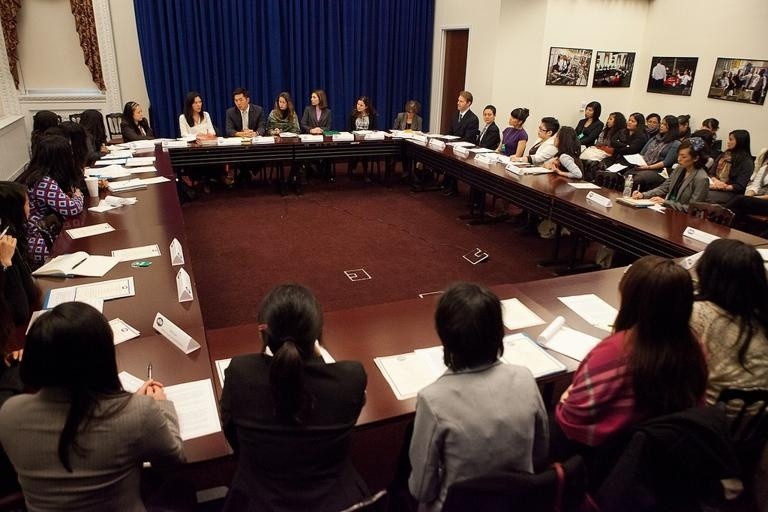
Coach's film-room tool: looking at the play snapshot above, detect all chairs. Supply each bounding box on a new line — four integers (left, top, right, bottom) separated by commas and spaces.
438, 455, 591, 512
104, 113, 123, 140
589, 401, 727, 512
706, 383, 766, 489
69, 113, 82, 125
684, 201, 736, 229
746, 214, 768, 224
321, 487, 402, 512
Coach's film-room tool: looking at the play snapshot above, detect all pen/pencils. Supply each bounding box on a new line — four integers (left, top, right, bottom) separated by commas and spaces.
72, 255, 89, 272
2, 224, 9, 238
147, 364, 156, 385
635, 185, 640, 197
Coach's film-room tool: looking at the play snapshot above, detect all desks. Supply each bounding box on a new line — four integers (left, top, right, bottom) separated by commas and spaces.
58, 147, 191, 232
31, 218, 234, 467
550, 188, 768, 279
108, 133, 294, 196
206, 280, 589, 467
405, 134, 495, 220
512, 243, 767, 413
289, 130, 422, 197
466, 155, 594, 266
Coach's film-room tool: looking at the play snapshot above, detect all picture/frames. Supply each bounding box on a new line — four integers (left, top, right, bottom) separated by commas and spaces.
646, 56, 699, 98
546, 46, 593, 86
707, 57, 768, 108
591, 50, 636, 88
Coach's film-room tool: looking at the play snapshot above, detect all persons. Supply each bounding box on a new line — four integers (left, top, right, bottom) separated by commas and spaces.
597, 112, 649, 191
496, 107, 530, 211
407, 283, 552, 512
22, 134, 85, 271
510, 116, 560, 234
707, 129, 754, 203
575, 101, 604, 148
347, 95, 379, 185
225, 87, 266, 191
219, 284, 373, 512
622, 114, 682, 191
555, 255, 711, 474
543, 126, 584, 179
645, 113, 661, 139
29, 110, 60, 161
0, 182, 37, 271
267, 92, 307, 198
721, 146, 768, 216
561, 54, 570, 70
677, 114, 692, 143
707, 57, 768, 106
674, 69, 693, 95
0, 347, 23, 368
585, 112, 627, 186
178, 90, 217, 196
300, 90, 336, 184
78, 108, 110, 157
650, 61, 668, 96
702, 118, 720, 142
439, 91, 480, 199
690, 129, 724, 176
48, 122, 90, 167
1, 301, 187, 512
632, 136, 710, 213
689, 238, 768, 420
468, 105, 501, 210
0, 226, 44, 326
120, 101, 156, 143
551, 55, 564, 76
393, 100, 422, 182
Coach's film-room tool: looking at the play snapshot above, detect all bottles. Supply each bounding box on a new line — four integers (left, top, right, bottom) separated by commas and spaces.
622, 174, 632, 199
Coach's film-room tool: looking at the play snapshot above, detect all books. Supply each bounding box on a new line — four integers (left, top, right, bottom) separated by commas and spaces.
32, 251, 118, 278
103, 180, 147, 195
